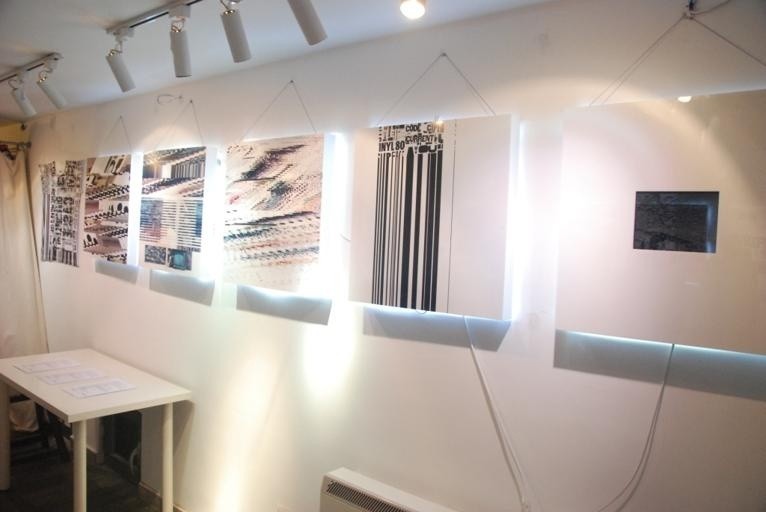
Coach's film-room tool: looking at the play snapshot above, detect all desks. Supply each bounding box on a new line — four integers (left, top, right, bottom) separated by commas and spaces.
0, 349, 190, 511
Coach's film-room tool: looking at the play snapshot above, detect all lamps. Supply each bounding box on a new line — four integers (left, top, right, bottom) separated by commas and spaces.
395, 0, 429, 21
99, 0, 329, 92
0, 51, 69, 119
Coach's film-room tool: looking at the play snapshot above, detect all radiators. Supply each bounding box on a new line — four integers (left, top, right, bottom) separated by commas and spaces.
317, 467, 457, 512
